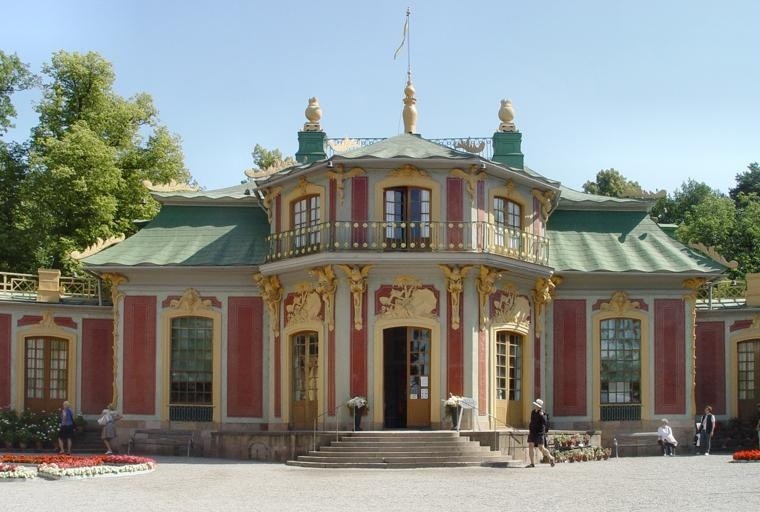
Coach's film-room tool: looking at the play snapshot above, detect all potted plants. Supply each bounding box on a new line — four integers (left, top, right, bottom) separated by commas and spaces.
553, 434, 611, 464
0, 408, 90, 452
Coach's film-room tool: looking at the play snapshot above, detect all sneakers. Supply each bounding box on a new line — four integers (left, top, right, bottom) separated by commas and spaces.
105, 451, 112, 455
550, 457, 555, 467
525, 464, 534, 468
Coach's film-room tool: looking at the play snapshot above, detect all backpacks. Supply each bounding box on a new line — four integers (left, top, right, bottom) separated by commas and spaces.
544, 412, 550, 433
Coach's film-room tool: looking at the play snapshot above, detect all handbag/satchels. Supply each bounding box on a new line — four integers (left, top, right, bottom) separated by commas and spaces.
59, 425, 77, 439
98, 416, 108, 426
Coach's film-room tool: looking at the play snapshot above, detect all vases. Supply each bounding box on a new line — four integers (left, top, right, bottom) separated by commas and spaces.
450, 405, 460, 430
352, 406, 362, 430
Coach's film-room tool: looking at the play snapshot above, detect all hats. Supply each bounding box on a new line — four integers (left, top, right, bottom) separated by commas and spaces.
533, 399, 544, 409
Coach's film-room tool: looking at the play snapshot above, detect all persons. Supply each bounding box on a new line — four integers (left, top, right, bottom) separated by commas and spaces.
97, 402, 117, 455
58, 401, 76, 455
655, 418, 676, 457
526, 398, 556, 468
697, 405, 715, 455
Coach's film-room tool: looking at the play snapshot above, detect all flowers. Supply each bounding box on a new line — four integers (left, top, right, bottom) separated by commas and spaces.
347, 396, 366, 407
440, 392, 461, 406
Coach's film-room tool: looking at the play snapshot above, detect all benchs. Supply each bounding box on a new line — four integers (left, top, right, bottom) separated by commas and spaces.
613, 432, 681, 458
128, 429, 195, 457
